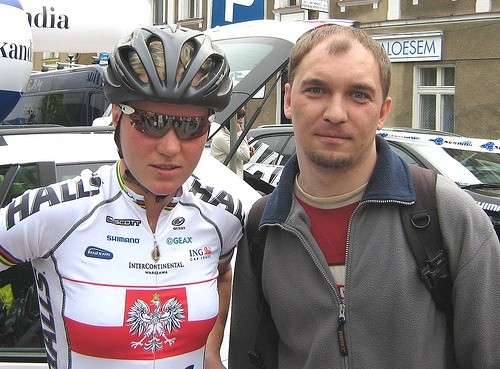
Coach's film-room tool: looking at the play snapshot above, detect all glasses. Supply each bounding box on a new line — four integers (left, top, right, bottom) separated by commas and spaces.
117, 103, 215, 141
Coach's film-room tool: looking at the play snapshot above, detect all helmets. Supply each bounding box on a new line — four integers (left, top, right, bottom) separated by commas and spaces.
104, 23, 232, 117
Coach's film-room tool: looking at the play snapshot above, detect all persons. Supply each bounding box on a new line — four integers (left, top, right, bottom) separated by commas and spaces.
227, 24, 500, 369
0, 24, 243, 369
210, 108, 256, 180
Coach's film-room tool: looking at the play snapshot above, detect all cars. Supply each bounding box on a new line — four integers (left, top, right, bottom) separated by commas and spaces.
246, 124, 500, 189
0, 18, 363, 368
1, 52, 111, 126
